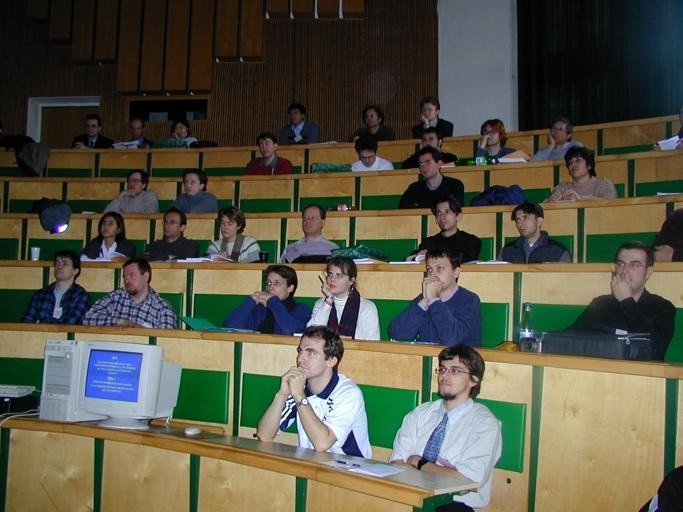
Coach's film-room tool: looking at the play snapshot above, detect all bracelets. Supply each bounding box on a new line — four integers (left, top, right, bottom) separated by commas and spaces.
324, 300, 332, 306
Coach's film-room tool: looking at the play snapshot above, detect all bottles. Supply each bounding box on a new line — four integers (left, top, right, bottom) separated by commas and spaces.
455, 157, 495, 165
520, 302, 534, 352
328, 203, 358, 210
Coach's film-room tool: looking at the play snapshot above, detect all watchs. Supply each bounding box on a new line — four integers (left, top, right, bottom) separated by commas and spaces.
296, 398, 309, 406
417, 456, 429, 470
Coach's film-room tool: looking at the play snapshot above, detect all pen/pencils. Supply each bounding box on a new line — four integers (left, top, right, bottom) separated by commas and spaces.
318, 275, 323, 283
334, 460, 360, 467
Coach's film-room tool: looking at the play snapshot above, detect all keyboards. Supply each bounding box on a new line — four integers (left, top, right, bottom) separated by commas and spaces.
0, 384, 36, 398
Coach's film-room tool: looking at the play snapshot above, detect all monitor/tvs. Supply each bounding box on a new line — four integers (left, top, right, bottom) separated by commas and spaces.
78, 341, 183, 430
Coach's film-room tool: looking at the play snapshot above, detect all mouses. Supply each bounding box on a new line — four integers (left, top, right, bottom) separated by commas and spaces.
184, 427, 203, 436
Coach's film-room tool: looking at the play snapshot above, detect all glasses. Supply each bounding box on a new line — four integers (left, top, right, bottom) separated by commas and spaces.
86, 125, 96, 128
265, 281, 281, 286
128, 179, 142, 183
435, 366, 472, 376
482, 129, 496, 134
614, 262, 644, 270
162, 220, 175, 225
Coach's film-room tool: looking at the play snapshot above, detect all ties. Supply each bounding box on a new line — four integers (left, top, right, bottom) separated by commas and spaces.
423, 413, 448, 463
90, 141, 94, 148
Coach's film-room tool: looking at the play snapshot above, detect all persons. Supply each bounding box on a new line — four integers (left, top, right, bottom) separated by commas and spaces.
71, 96, 682, 262
79, 257, 178, 329
566, 241, 677, 362
257, 325, 372, 459
386, 238, 483, 347
306, 256, 380, 341
389, 342, 502, 512
20, 250, 90, 324
223, 264, 312, 335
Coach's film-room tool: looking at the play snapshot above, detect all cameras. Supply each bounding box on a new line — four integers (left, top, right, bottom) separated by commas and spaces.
519, 337, 542, 353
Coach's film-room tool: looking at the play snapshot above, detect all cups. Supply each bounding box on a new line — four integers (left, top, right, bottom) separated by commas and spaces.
30, 248, 40, 261
257, 252, 270, 263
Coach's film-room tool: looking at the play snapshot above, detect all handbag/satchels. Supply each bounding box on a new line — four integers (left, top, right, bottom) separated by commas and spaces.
331, 244, 388, 261
542, 327, 653, 360
470, 184, 527, 205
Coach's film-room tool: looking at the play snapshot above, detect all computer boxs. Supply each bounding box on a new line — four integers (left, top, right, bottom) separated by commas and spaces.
39, 339, 109, 422
